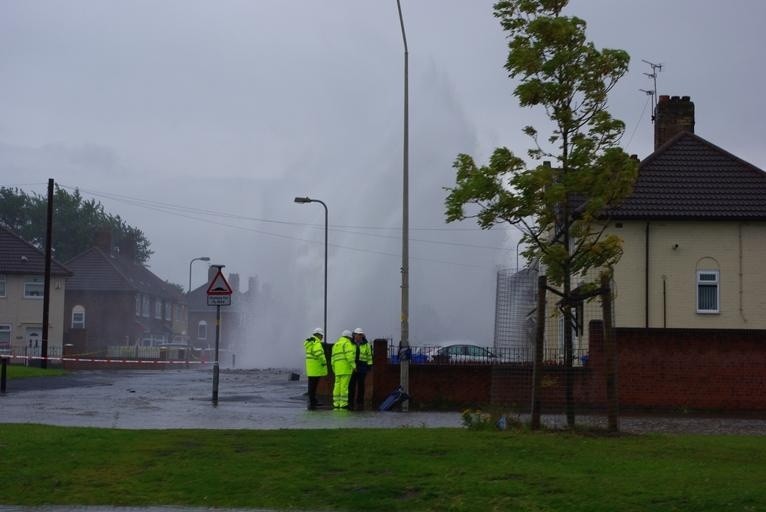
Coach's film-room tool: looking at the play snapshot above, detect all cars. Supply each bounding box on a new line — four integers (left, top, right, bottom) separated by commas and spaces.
422, 343, 517, 363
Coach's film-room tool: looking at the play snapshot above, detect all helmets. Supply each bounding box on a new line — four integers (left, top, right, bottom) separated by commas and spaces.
312, 328, 324, 336
342, 330, 353, 338
354, 328, 364, 334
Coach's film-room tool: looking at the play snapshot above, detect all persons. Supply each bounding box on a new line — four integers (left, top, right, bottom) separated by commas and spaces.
331, 329, 358, 409
303, 328, 328, 411
348, 328, 373, 411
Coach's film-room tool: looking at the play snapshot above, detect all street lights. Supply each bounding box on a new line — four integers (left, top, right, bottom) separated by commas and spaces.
185, 255, 210, 368
295, 197, 328, 347
